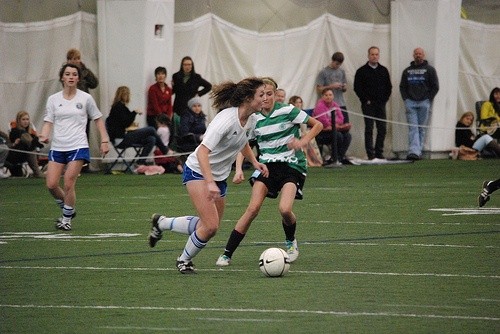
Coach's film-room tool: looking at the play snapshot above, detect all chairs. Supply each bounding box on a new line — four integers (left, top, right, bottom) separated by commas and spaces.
304, 109, 337, 164
169, 116, 197, 166
105, 117, 144, 176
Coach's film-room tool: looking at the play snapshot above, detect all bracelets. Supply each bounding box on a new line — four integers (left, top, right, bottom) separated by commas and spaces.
101, 142, 109, 143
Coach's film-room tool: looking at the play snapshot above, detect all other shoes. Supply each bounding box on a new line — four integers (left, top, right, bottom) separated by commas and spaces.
56, 212, 78, 229
61, 217, 73, 231
407, 154, 419, 160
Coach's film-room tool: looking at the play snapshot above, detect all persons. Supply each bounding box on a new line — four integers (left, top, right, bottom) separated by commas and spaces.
38, 63, 109, 230
456, 87, 500, 158
67, 48, 99, 172
477, 178, 500, 207
215, 76, 323, 265
353, 47, 393, 159
315, 52, 349, 123
4, 110, 49, 177
147, 56, 212, 161
148, 77, 269, 274
399, 48, 440, 160
274, 88, 351, 165
109, 86, 183, 174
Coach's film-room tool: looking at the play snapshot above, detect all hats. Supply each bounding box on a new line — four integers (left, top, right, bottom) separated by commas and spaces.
187, 96, 202, 109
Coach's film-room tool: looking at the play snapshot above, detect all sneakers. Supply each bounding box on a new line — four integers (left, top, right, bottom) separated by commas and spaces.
478, 180, 494, 207
176, 252, 196, 273
148, 213, 163, 247
285, 237, 299, 263
216, 253, 231, 266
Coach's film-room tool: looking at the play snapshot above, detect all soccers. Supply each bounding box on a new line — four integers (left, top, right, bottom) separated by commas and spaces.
259, 248, 290, 277
